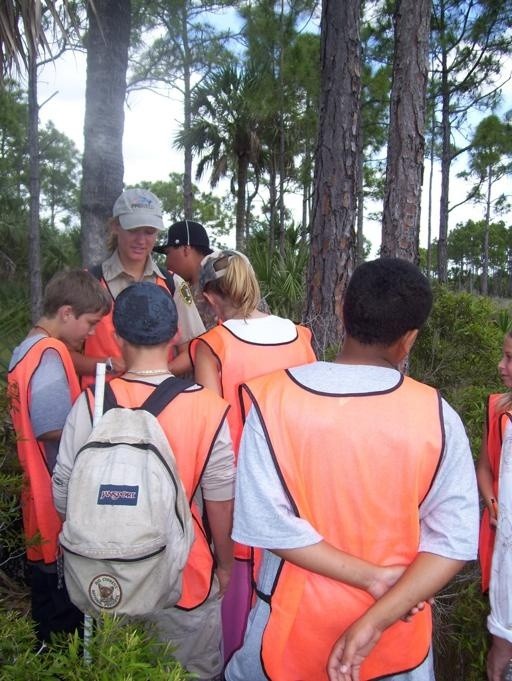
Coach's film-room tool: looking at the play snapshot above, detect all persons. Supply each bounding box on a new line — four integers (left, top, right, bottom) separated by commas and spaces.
188, 246, 317, 590
50, 281, 235, 680
223, 256, 486, 681
150, 218, 268, 329
9, 270, 111, 648
63, 189, 206, 390
475, 408, 512, 681
479, 332, 512, 594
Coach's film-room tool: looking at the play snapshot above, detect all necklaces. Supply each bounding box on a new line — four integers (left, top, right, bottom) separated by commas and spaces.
127, 369, 172, 376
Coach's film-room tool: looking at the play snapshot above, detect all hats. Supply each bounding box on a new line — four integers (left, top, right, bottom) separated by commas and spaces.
112, 188, 165, 231
153, 220, 209, 254
113, 282, 178, 344
199, 249, 250, 291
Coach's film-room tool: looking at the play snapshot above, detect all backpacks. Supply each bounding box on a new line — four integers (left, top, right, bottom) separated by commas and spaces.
57, 378, 195, 619
